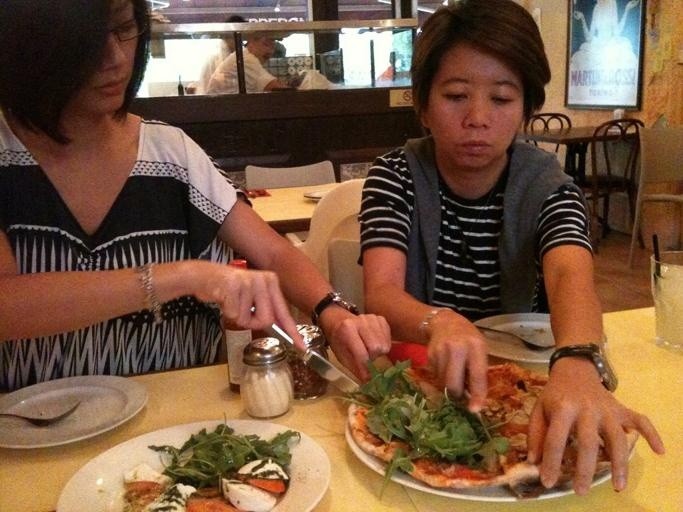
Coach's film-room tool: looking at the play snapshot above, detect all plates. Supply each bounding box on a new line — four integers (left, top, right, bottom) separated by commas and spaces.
301, 188, 329, 202
472, 311, 612, 365
3, 375, 146, 449
341, 421, 639, 504
47, 420, 331, 511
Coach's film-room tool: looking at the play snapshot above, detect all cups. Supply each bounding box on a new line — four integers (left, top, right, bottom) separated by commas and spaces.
648, 250, 681, 350
611, 109, 626, 129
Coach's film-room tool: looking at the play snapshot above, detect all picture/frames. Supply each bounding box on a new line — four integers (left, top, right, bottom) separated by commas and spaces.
562, 0, 647, 113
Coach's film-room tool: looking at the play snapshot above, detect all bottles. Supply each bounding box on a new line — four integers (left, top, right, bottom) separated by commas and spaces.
224, 258, 269, 391
284, 326, 330, 399
239, 339, 292, 420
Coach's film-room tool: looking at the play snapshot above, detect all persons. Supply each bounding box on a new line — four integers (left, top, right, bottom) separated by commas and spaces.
204, 30, 296, 92
196, 13, 247, 93
355, 2, 668, 498
0, 2, 393, 395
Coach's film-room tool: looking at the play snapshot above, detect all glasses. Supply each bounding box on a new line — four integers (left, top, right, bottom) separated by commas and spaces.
111, 15, 147, 42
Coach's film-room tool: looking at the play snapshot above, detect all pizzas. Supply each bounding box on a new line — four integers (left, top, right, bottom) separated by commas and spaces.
347, 361, 639, 488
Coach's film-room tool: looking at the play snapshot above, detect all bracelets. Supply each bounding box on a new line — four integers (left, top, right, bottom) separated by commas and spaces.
136, 259, 163, 311
415, 305, 452, 342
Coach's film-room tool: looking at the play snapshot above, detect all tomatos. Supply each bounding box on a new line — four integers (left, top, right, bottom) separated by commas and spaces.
185, 471, 285, 512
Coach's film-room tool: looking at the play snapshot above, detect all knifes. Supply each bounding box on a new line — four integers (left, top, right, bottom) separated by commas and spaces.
271, 327, 368, 398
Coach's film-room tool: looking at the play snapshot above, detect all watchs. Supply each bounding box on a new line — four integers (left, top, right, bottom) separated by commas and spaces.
311, 287, 360, 330
546, 341, 620, 391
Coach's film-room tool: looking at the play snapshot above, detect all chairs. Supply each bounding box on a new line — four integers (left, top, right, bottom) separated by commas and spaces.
627, 122, 683, 270
318, 231, 371, 316
521, 111, 574, 154
578, 117, 647, 257
282, 172, 371, 326
241, 159, 338, 193
